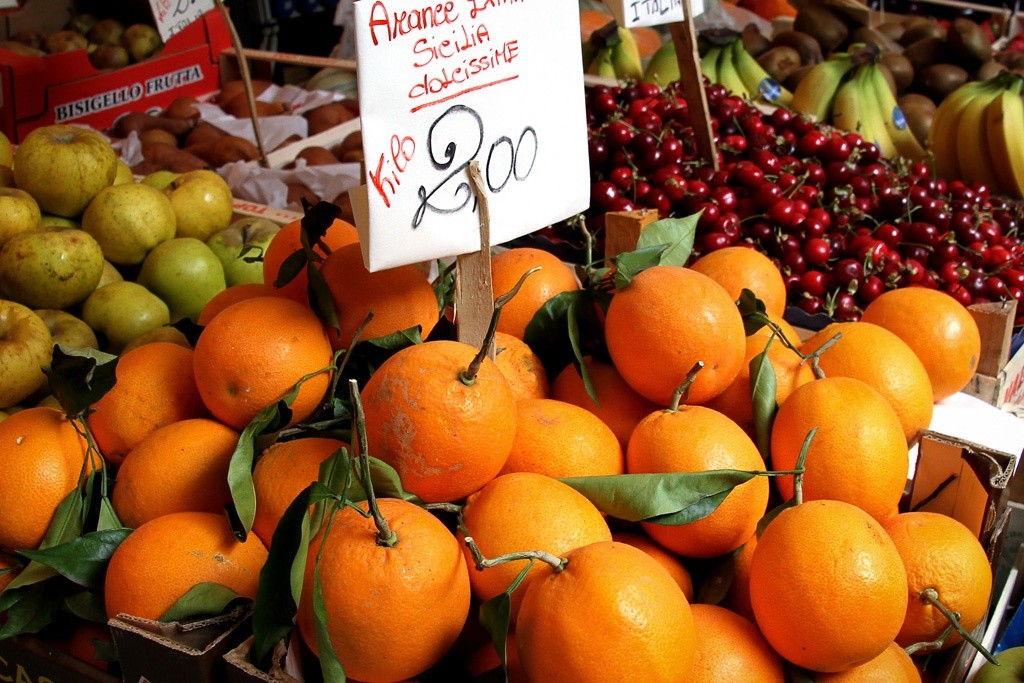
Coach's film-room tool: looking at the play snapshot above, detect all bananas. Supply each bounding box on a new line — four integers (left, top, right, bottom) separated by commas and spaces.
571, 8, 1024, 200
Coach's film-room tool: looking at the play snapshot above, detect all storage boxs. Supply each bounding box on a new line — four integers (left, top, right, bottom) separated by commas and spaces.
213, 111, 362, 228
0, 1, 234, 150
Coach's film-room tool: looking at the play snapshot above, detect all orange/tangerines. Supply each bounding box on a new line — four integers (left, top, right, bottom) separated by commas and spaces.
2, 208, 1010, 680
